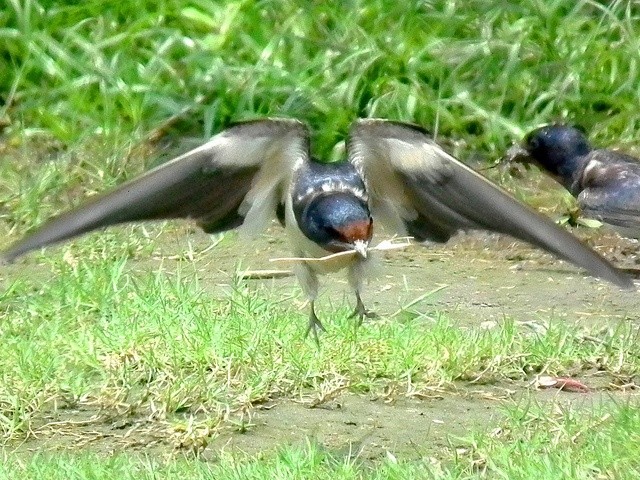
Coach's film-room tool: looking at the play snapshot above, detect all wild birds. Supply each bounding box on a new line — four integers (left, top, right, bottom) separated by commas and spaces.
0, 115, 635, 351
514, 123, 640, 241
